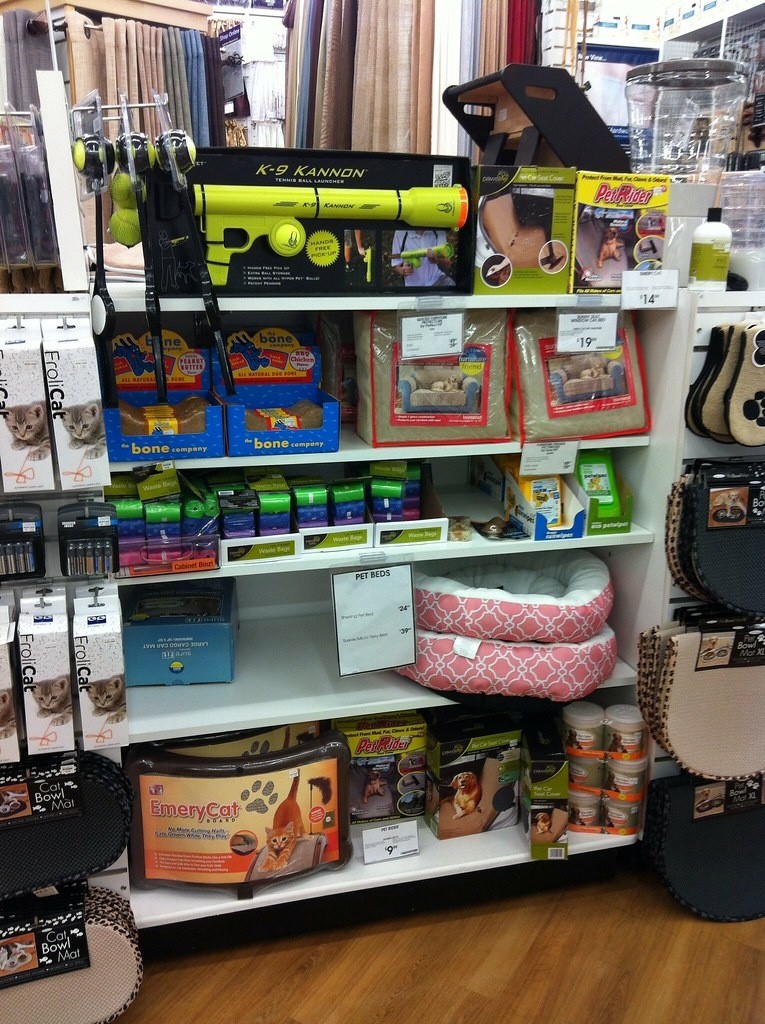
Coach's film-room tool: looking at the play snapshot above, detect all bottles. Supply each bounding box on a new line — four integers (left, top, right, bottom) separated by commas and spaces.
688, 207, 733, 292
562, 693, 646, 834
718, 160, 765, 292
625, 55, 748, 191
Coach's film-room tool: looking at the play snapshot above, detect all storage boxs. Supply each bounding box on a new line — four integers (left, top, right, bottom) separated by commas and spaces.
121, 577, 238, 687
469, 455, 632, 541
0, 584, 128, 765
118, 477, 449, 575
0, 317, 341, 493
330, 717, 570, 861
145, 146, 476, 298
461, 165, 670, 296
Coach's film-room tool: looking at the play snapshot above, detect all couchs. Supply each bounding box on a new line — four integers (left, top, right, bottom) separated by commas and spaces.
397, 369, 478, 412
549, 357, 622, 404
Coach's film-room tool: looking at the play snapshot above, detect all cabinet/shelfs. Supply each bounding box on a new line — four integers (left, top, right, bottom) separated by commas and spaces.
86, 283, 765, 928
